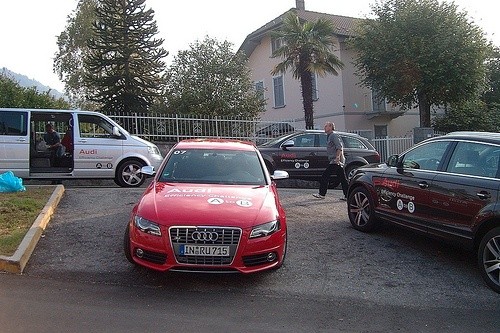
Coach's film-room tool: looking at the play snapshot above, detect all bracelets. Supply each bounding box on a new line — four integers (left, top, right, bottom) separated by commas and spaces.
49, 145, 50, 149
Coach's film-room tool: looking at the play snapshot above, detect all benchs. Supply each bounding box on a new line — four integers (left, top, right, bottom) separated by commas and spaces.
30, 131, 53, 167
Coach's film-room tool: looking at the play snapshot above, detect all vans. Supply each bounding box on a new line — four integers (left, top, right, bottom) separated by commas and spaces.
0, 107, 164, 187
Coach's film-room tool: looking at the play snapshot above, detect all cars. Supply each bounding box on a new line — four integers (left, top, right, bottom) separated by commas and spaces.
123, 137, 288, 279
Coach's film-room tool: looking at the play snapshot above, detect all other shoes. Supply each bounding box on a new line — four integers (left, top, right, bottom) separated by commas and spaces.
340, 195, 347, 200
313, 193, 325, 199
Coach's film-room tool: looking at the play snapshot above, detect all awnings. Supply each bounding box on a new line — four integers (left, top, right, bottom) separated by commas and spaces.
255, 123, 294, 135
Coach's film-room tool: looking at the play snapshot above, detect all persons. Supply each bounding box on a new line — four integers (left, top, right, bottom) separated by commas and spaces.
312, 122, 352, 201
39, 124, 62, 168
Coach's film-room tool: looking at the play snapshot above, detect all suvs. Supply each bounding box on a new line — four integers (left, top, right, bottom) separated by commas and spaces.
256, 130, 382, 190
343, 131, 500, 292
